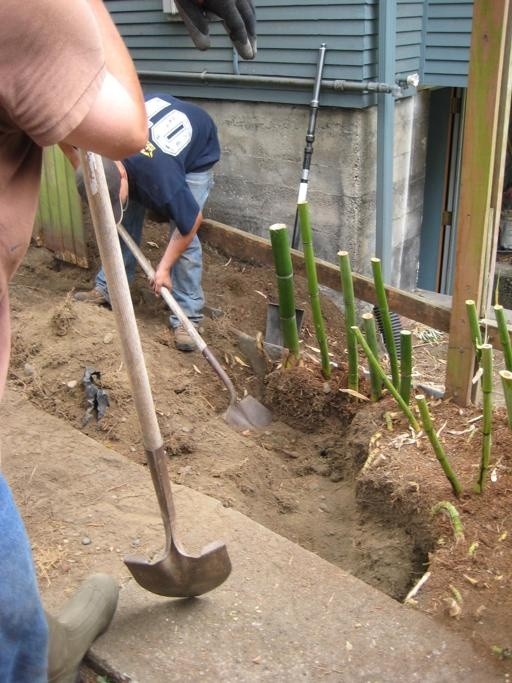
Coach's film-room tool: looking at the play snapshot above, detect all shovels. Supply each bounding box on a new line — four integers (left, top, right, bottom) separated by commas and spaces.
77, 146, 232, 598
115, 223, 274, 432
265, 43, 327, 350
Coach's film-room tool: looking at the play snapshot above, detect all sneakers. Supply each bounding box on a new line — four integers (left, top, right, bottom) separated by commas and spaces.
174, 322, 198, 351
75, 288, 105, 304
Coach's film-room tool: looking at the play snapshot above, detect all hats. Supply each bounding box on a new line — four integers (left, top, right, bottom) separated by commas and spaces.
76, 157, 123, 226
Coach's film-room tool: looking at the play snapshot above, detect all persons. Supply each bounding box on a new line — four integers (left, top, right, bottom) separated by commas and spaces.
75, 93, 222, 355
1, 0, 260, 683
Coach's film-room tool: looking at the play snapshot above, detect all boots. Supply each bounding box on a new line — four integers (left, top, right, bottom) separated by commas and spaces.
43, 574, 117, 681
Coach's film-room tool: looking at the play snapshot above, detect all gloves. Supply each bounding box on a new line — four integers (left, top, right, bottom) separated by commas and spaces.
173, 0, 257, 59
